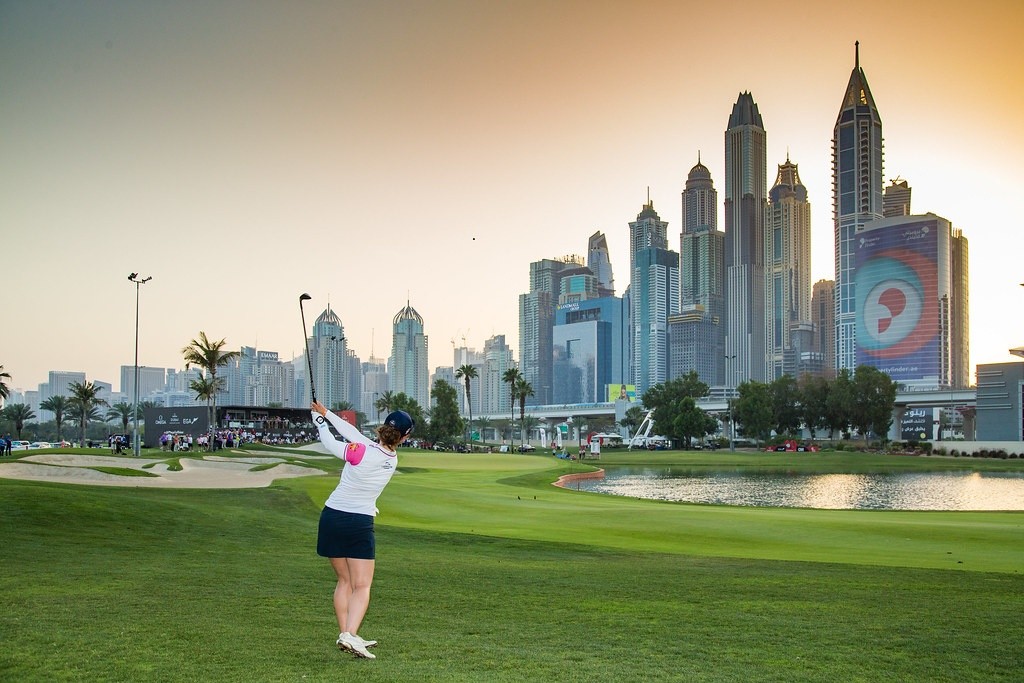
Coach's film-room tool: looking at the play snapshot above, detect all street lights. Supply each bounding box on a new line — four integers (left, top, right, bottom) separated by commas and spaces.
127, 273, 153, 457
331, 336, 346, 412
724, 354, 737, 451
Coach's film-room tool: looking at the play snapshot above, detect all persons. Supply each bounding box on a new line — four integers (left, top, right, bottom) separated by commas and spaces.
398, 438, 433, 450
579, 449, 585, 459
88, 441, 92, 448
80, 439, 84, 447
312, 401, 415, 659
341, 436, 350, 442
109, 433, 129, 454
370, 435, 378, 442
647, 441, 660, 450
566, 452, 570, 458
552, 448, 556, 457
160, 413, 320, 453
0, 432, 13, 456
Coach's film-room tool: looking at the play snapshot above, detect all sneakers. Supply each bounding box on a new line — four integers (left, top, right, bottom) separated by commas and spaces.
335, 632, 378, 649
338, 632, 376, 659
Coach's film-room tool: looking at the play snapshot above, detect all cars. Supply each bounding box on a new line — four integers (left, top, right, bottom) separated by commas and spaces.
4, 440, 62, 450
693, 440, 720, 450
518, 444, 536, 453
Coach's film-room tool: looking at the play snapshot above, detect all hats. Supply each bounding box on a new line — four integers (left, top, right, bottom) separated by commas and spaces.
384, 410, 416, 438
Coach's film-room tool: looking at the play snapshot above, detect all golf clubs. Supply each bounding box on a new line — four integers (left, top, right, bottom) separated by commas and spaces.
298, 294, 317, 402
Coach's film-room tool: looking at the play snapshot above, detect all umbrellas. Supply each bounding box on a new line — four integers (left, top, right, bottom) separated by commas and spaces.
635, 434, 679, 441
